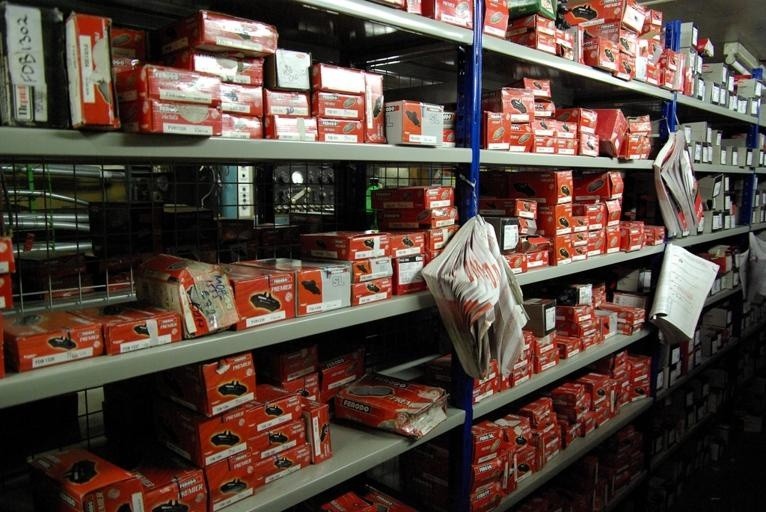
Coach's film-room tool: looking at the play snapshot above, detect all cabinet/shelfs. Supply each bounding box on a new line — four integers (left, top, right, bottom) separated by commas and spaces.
4, 1, 762, 508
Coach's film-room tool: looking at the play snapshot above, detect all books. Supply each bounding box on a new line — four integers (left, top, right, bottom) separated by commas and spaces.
652, 129, 703, 235
422, 214, 528, 381
738, 231, 766, 302
648, 243, 720, 348
